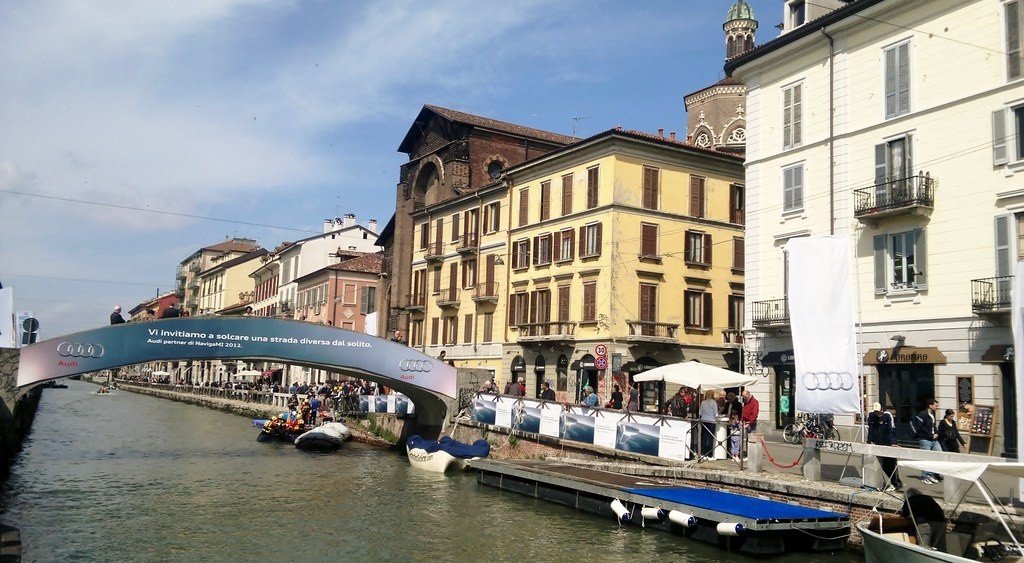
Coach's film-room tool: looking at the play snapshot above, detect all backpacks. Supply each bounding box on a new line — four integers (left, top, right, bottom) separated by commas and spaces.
907, 415, 923, 438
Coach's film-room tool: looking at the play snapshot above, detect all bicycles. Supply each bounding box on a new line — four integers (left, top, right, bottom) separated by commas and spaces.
783, 417, 841, 445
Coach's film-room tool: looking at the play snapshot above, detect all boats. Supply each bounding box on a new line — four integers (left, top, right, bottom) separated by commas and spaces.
854, 460, 1024, 563
256, 419, 491, 473
96, 386, 116, 394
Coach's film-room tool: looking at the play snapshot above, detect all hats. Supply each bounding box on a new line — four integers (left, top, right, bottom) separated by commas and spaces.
719, 390, 726, 398
873, 402, 883, 412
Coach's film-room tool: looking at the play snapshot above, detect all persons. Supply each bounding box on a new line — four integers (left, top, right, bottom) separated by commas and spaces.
478, 376, 640, 413
914, 399, 943, 485
867, 402, 903, 493
662, 387, 759, 461
901, 489, 947, 552
937, 409, 967, 453
110, 306, 456, 425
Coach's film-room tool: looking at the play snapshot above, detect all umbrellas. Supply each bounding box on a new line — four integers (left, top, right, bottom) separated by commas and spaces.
633, 359, 758, 418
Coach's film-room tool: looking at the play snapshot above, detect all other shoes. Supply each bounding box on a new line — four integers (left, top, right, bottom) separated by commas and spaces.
732, 457, 740, 463
703, 456, 716, 461
922, 476, 939, 485
886, 484, 903, 492
